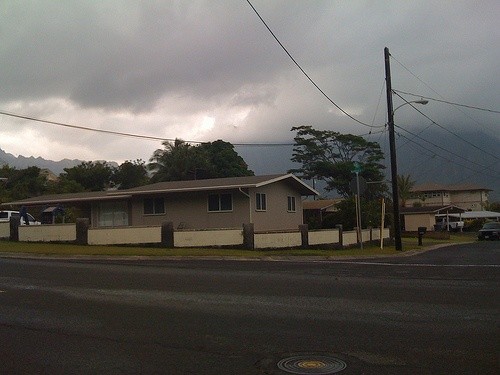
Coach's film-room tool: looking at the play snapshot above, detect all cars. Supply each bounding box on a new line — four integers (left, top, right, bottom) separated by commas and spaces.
477, 222, 500, 241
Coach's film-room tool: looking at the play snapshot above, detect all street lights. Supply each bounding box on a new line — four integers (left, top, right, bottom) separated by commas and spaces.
388, 99, 429, 251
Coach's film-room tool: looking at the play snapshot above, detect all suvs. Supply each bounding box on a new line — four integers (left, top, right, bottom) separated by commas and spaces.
0, 211, 41, 227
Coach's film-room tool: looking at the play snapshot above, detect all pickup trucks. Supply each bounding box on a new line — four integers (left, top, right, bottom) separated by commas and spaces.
436, 217, 465, 232
